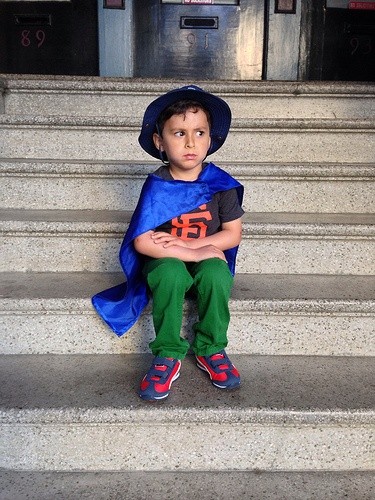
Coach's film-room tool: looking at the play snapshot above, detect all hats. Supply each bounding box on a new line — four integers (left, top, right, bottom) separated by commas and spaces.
138, 84, 231, 161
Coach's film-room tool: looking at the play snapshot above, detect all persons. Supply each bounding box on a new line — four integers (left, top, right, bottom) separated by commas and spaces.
91, 85, 245, 400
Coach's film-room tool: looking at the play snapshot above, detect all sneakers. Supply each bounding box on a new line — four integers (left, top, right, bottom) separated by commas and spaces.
195, 348, 241, 389
138, 356, 182, 400
